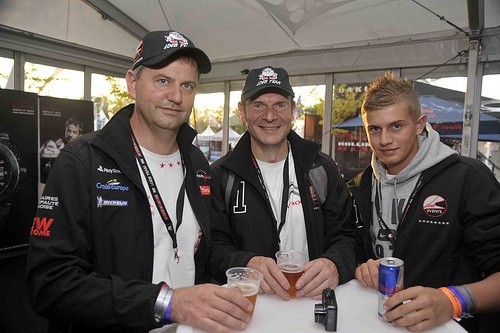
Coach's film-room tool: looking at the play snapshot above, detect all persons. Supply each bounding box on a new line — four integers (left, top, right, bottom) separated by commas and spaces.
347, 71, 500, 333
23, 29, 253, 333
40, 116, 84, 182
208, 65, 359, 300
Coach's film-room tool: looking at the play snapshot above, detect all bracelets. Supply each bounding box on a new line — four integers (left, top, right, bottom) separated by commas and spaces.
438, 284, 477, 322
153, 283, 175, 323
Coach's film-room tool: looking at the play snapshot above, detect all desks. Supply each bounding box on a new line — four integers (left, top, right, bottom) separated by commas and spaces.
174, 279, 471, 333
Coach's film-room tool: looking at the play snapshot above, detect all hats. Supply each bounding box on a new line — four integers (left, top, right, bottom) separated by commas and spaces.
241, 66, 295, 102
133, 30, 211, 74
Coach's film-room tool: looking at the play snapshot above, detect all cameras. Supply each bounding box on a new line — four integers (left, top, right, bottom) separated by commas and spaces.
314, 287, 337, 331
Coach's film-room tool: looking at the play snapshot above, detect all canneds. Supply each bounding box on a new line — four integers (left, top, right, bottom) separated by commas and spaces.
378, 257, 405, 319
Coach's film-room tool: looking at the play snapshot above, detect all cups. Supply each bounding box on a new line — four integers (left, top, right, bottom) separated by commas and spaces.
225, 266, 265, 318
275, 250, 308, 300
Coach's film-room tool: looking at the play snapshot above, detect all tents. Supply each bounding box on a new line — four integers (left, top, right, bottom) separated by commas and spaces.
193, 125, 240, 151
332, 95, 500, 164
